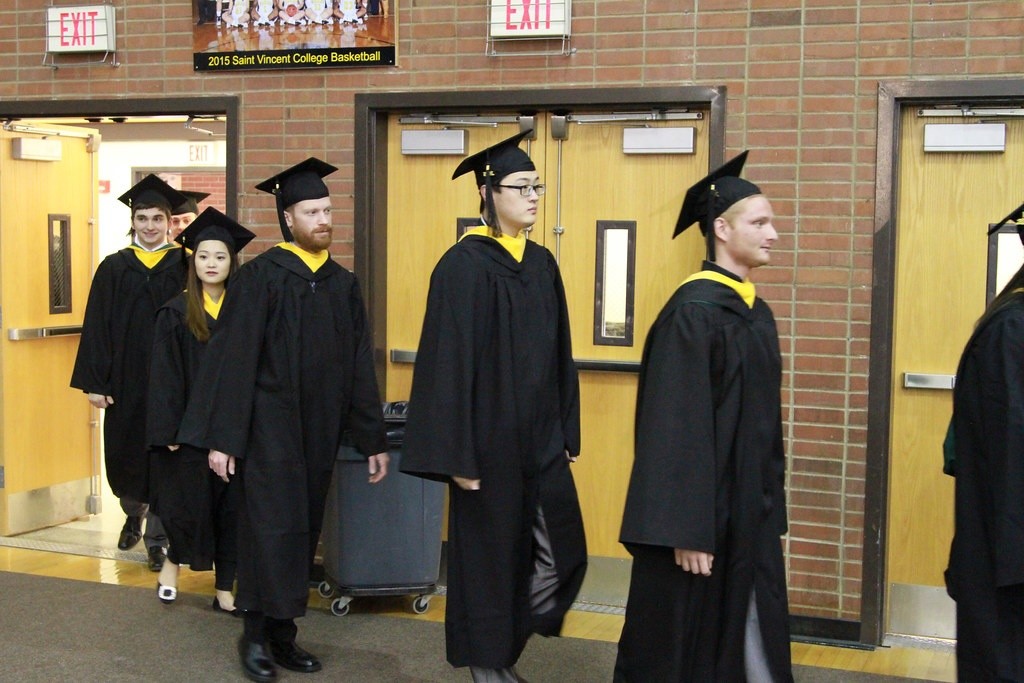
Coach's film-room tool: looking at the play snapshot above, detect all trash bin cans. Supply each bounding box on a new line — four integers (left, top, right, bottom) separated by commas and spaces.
328, 402, 446, 616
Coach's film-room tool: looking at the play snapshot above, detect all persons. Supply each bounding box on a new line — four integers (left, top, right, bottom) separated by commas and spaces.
943, 203, 1024, 683
398, 129, 587, 683
196, 0, 368, 29
612, 150, 795, 683
70, 173, 211, 573
178, 157, 390, 683
145, 206, 257, 618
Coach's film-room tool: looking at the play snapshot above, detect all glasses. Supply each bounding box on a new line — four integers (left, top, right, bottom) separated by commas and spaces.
495, 184, 546, 196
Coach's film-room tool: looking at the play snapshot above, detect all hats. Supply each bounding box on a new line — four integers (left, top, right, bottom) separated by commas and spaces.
254, 157, 338, 242
170, 190, 211, 216
452, 128, 537, 237
116, 173, 188, 212
987, 202, 1024, 245
173, 206, 256, 292
671, 149, 763, 262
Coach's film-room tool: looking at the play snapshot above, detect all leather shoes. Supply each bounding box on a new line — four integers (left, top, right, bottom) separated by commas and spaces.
268, 640, 321, 672
148, 545, 166, 571
211, 596, 248, 617
118, 514, 144, 551
156, 581, 178, 604
237, 634, 277, 682
